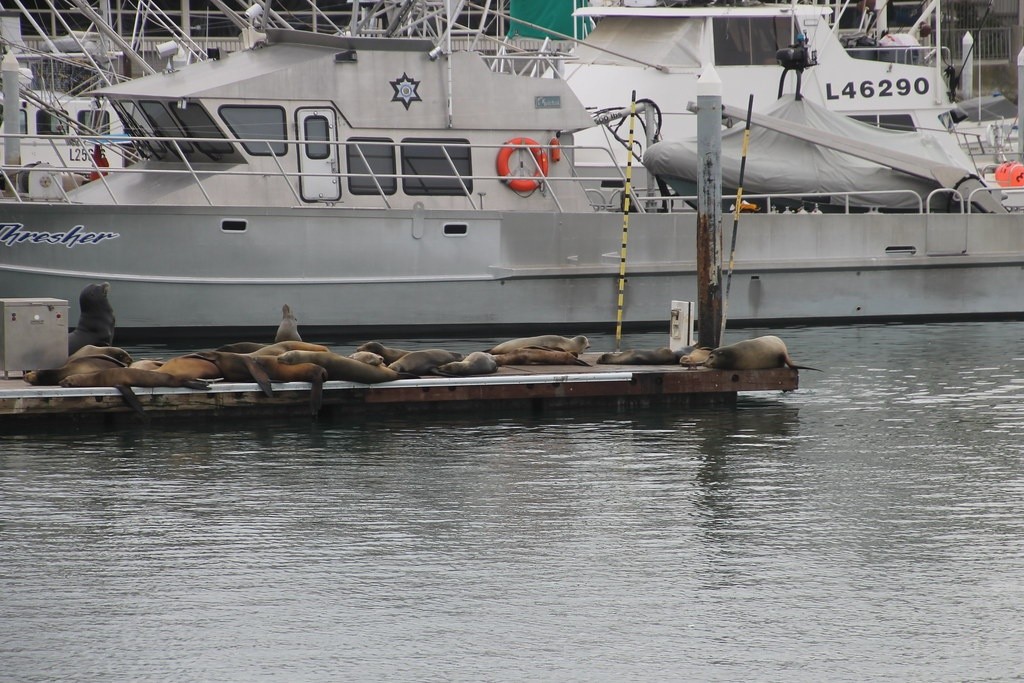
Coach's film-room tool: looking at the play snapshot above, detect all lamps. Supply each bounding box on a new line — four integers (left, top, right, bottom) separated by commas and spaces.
695, 62, 722, 96
155, 40, 180, 73
244, 2, 263, 29
428, 47, 447, 62
1, 50, 19, 71
177, 99, 187, 111
962, 31, 973, 45
92, 98, 101, 110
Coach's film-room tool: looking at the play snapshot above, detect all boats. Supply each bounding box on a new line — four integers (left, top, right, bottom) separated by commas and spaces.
0, 0, 1024, 340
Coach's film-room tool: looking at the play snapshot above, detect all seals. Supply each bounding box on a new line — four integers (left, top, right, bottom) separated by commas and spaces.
23, 280, 594, 416
597, 335, 825, 375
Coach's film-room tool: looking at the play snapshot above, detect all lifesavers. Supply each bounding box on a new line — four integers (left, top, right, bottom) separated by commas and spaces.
498, 137, 549, 193
